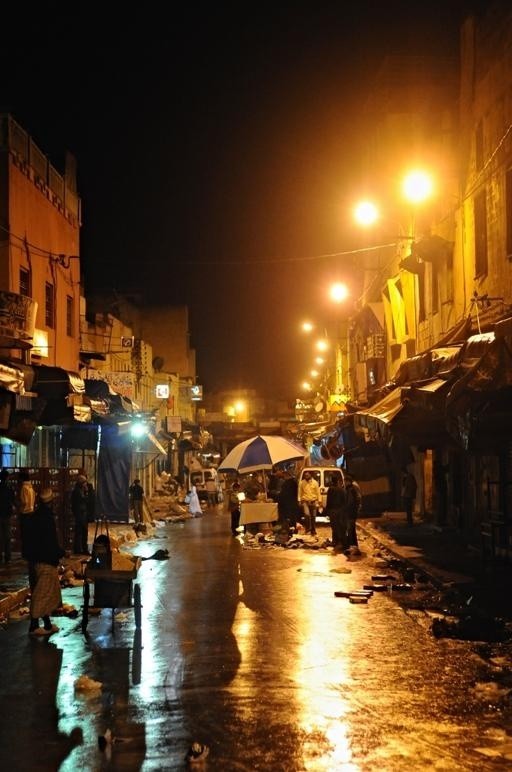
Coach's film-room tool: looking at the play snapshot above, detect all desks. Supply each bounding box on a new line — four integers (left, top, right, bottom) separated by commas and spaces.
237, 499, 284, 536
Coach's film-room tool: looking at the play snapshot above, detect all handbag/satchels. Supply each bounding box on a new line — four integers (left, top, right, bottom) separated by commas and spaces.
92, 535, 110, 568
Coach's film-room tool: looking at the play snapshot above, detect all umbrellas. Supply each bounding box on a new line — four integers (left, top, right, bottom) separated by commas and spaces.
216, 433, 308, 502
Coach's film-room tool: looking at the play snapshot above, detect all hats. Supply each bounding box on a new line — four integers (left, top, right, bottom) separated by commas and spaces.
78, 475, 87, 484
39, 488, 54, 503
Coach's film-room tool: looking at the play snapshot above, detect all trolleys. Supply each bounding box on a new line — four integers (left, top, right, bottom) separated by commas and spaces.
78, 514, 143, 635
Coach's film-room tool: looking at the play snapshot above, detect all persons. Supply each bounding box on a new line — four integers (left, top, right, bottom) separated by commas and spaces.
397, 466, 418, 527
1, 470, 21, 564
85, 483, 96, 523
226, 470, 363, 557
69, 475, 93, 555
129, 479, 146, 522
14, 471, 38, 559
22, 487, 73, 639
429, 448, 453, 528
158, 465, 220, 518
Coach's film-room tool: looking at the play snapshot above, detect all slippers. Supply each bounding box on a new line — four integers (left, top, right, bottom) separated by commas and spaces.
28, 624, 58, 634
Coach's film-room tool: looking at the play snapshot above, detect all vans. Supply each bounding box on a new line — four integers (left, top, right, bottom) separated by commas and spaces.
188, 468, 222, 504
297, 466, 347, 514
